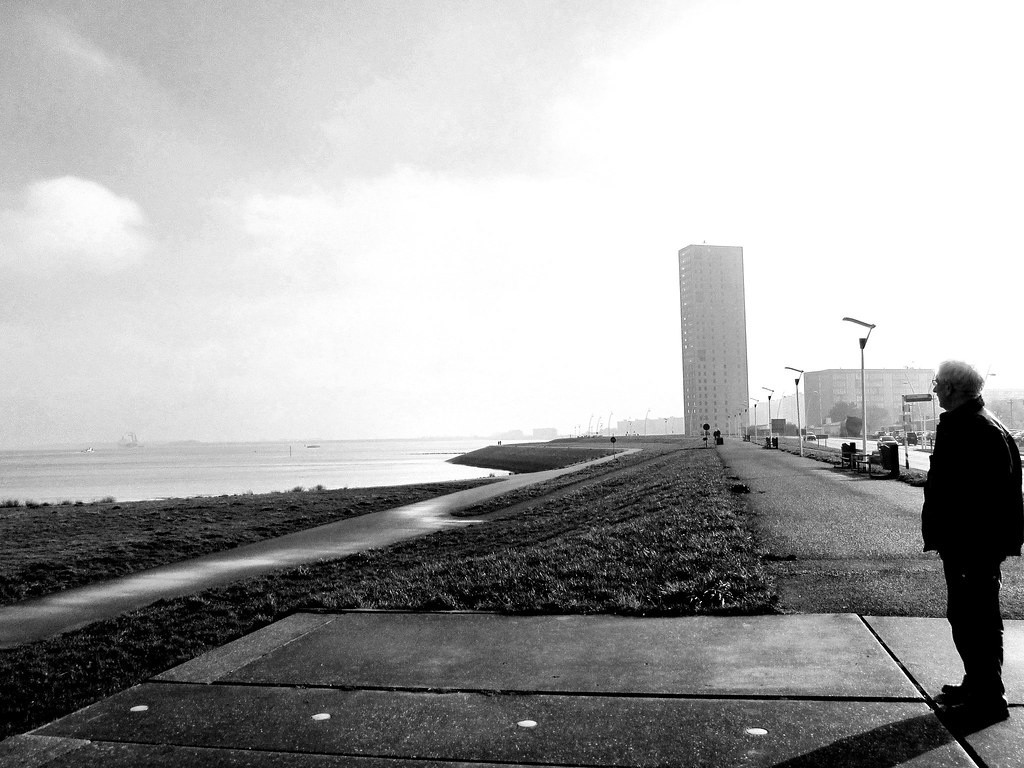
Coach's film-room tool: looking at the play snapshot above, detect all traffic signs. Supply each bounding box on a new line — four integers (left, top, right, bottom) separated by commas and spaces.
905, 394, 932, 403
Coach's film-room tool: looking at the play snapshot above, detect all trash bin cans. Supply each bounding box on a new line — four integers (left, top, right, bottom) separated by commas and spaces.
880, 444, 899, 474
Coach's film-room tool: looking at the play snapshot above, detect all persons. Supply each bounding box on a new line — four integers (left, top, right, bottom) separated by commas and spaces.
921, 361, 1024, 722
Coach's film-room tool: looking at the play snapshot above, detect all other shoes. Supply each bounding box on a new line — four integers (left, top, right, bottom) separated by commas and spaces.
942, 681, 976, 697
947, 697, 1010, 720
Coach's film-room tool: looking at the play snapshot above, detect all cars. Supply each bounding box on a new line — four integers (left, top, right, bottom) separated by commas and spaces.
892, 429, 937, 446
872, 431, 886, 439
877, 436, 899, 451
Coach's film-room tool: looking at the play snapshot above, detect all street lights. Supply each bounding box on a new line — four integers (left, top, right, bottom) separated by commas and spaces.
750, 398, 760, 444
841, 316, 877, 471
762, 387, 774, 447
784, 366, 804, 458
727, 402, 750, 440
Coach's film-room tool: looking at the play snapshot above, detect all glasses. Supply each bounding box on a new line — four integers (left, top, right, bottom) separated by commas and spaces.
932, 379, 942, 386
